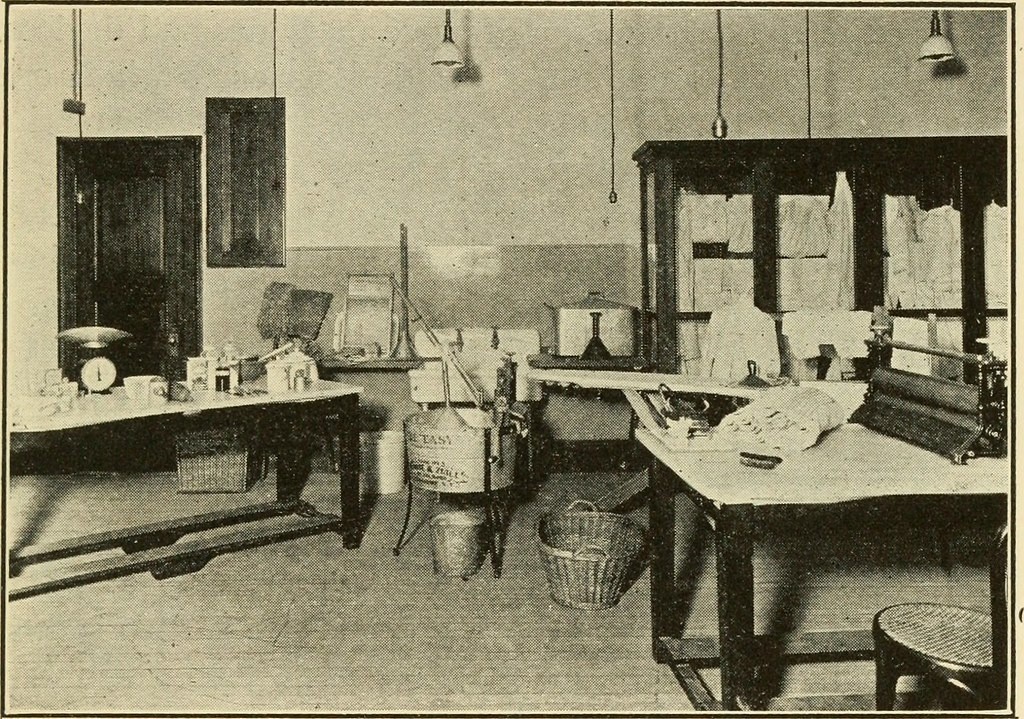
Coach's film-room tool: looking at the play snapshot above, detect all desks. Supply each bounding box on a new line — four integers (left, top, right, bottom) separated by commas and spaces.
9, 352, 1007, 711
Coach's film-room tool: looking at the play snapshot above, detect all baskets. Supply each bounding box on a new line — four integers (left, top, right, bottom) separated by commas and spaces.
174, 426, 250, 494
533, 498, 644, 610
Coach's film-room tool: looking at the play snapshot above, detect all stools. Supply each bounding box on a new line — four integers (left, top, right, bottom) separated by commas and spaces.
871, 601, 1008, 710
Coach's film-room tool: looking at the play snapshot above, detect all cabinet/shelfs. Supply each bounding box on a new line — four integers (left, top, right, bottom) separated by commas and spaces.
633, 134, 1008, 374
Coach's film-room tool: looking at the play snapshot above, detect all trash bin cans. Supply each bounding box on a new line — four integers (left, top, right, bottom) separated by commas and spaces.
431, 510, 484, 578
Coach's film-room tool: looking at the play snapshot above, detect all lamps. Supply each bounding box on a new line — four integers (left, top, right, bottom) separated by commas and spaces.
917, 10, 957, 62
431, 8, 464, 70
